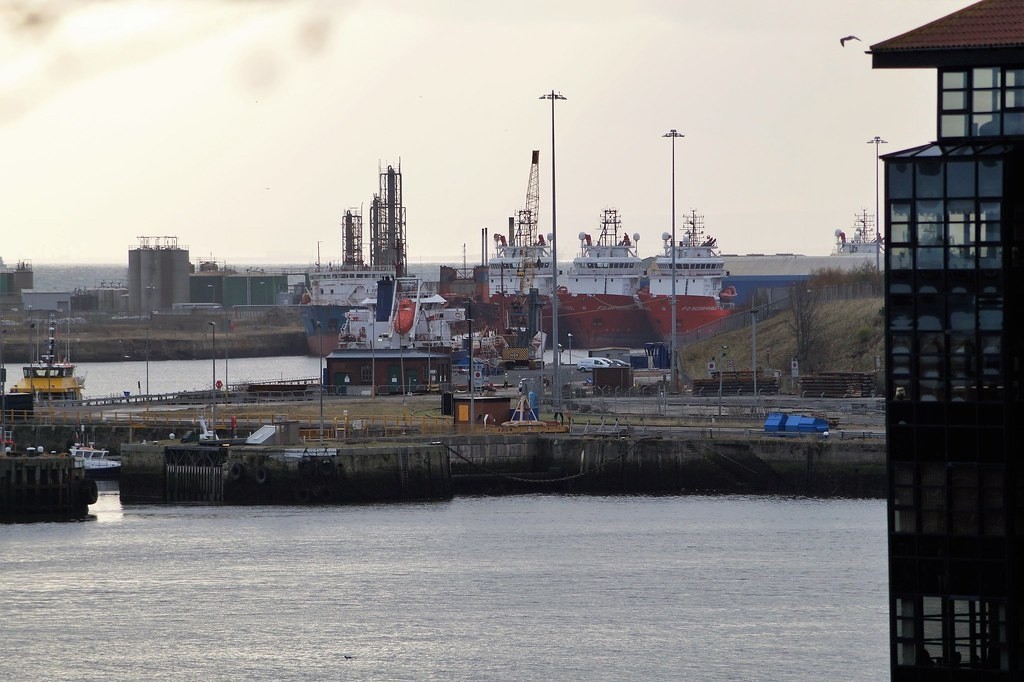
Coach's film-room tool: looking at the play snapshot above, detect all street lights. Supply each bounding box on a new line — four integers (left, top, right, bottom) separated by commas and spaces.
146, 308, 160, 401
314, 320, 325, 446
208, 320, 219, 442
661, 125, 686, 394
867, 133, 887, 271
750, 308, 759, 404
568, 333, 572, 365
539, 88, 568, 412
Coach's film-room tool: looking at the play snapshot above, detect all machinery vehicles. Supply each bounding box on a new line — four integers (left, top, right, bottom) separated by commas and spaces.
764, 411, 831, 437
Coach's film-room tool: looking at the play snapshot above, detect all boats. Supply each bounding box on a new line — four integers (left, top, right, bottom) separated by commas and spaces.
639, 210, 740, 341
482, 231, 559, 343
7, 305, 84, 405
331, 294, 469, 353
67, 443, 122, 478
297, 266, 448, 356
553, 205, 660, 349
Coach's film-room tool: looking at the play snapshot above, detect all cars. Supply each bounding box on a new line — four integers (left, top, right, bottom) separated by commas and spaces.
596, 357, 623, 368
519, 377, 535, 391
612, 359, 632, 367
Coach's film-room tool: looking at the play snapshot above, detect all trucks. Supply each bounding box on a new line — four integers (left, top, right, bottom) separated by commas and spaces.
577, 358, 611, 373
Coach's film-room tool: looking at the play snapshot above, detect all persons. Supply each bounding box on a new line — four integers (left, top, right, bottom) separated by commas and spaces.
504, 373, 509, 387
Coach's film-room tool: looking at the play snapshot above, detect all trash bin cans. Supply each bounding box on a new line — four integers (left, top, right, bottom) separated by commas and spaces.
785, 414, 802, 432
764, 412, 788, 432
799, 416, 830, 433
